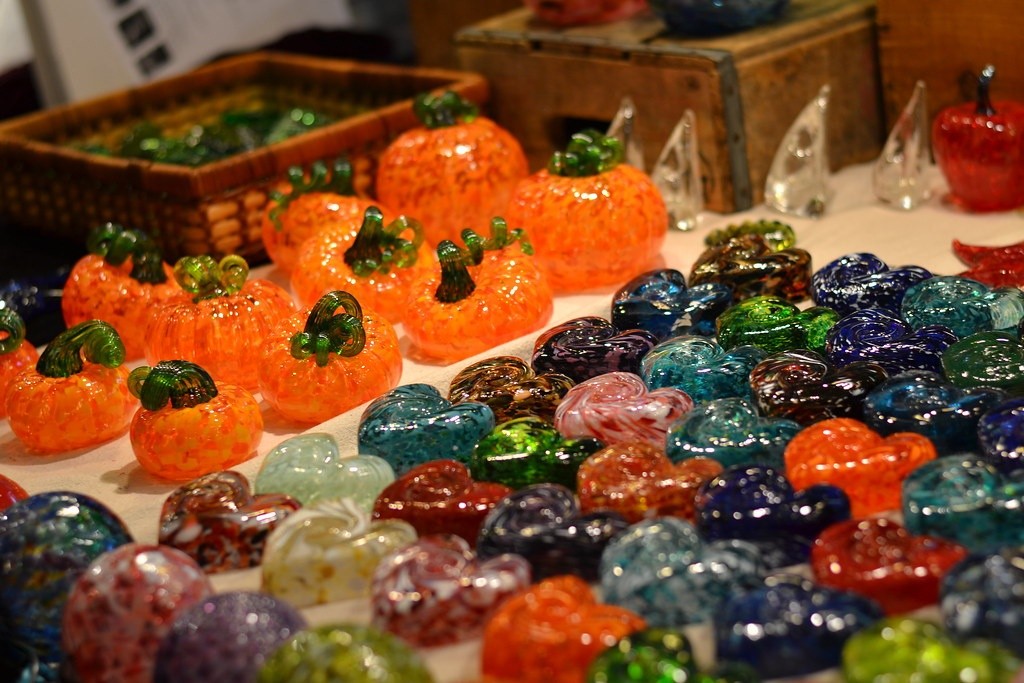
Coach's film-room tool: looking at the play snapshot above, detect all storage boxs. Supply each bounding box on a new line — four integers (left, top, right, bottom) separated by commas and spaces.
0, 0, 886, 271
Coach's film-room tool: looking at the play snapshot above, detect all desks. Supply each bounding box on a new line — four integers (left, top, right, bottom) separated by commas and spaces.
0, 159, 1024, 683
